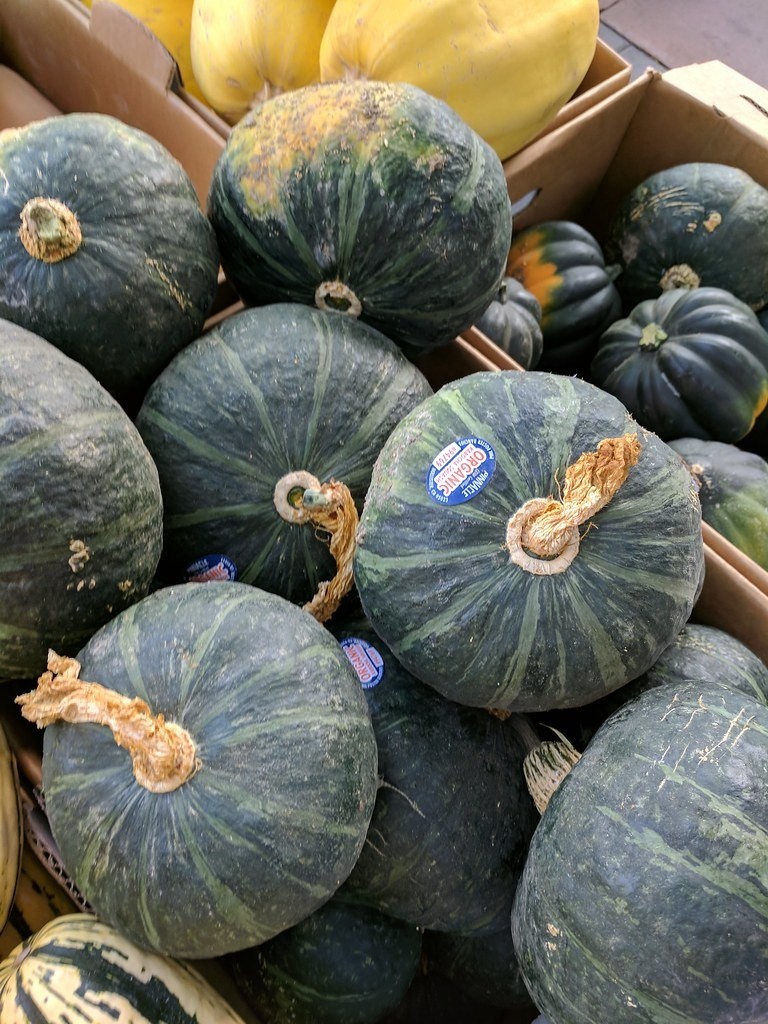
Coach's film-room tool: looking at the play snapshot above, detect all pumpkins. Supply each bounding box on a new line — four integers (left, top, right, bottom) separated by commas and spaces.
0, 0, 768, 1024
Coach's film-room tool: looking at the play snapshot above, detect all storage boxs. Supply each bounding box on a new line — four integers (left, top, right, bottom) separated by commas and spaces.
0, 0, 768, 914
